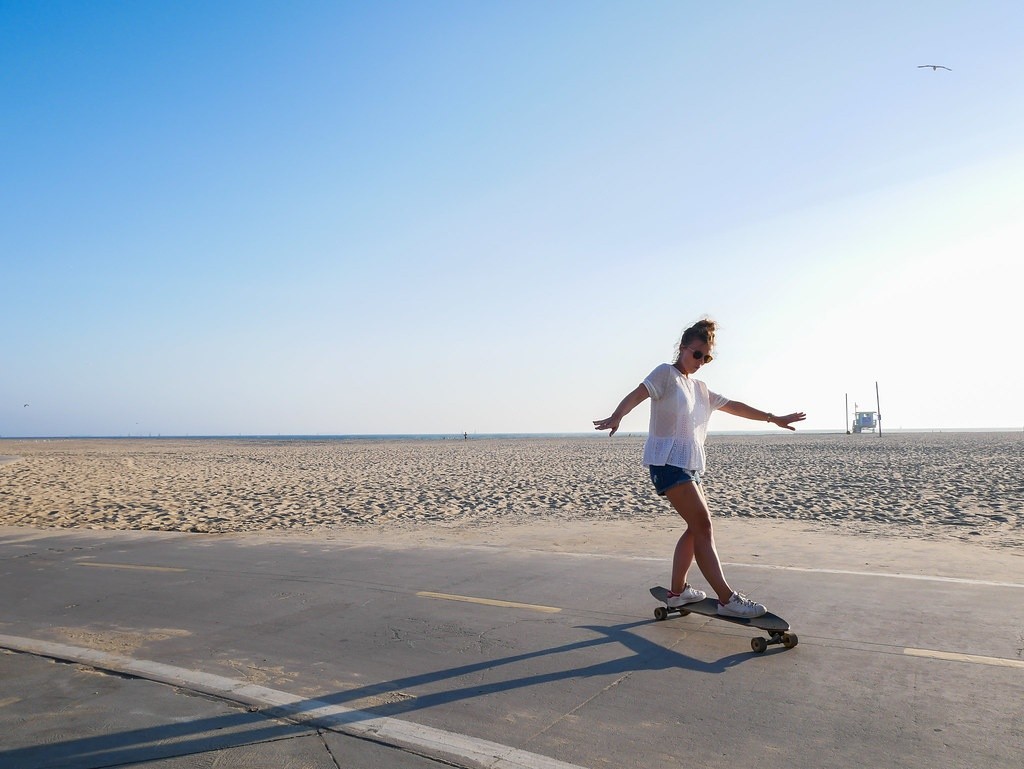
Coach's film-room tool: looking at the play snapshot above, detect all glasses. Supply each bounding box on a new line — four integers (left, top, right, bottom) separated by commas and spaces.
686, 346, 713, 363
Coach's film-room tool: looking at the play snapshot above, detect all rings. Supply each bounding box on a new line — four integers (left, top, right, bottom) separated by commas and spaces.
604, 423, 606, 426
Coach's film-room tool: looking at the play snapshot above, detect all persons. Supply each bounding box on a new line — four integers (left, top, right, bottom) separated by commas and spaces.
593, 319, 806, 618
464, 432, 467, 442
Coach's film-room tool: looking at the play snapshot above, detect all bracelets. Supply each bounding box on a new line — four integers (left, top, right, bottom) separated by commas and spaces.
767, 413, 772, 423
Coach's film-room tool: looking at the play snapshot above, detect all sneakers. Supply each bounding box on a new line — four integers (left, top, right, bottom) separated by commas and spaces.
717, 591, 767, 619
667, 583, 706, 608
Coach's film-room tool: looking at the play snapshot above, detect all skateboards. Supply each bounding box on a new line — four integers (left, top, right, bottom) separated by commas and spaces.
649, 585, 799, 653
